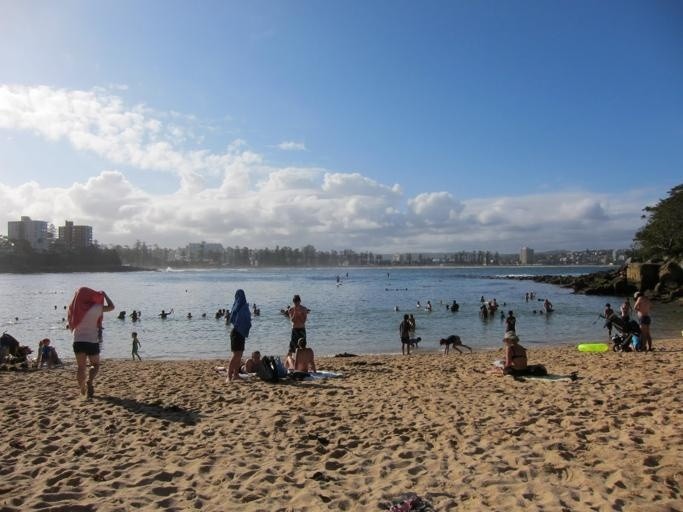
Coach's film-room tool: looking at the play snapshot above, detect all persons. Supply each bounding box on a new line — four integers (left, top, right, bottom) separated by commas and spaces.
213, 289, 318, 384
398, 313, 422, 356
497, 310, 528, 377
132, 332, 142, 362
439, 334, 472, 355
604, 291, 651, 353
119, 310, 142, 322
416, 290, 556, 320
68, 289, 115, 397
33, 338, 62, 367
159, 310, 172, 320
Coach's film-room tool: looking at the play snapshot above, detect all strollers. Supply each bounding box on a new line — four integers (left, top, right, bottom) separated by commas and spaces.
599, 313, 643, 352
0, 333, 31, 364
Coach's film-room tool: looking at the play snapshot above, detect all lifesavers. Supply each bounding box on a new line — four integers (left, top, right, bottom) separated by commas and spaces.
578, 343, 609, 353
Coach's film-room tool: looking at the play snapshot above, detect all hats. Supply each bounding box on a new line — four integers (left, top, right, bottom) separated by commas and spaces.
501, 330, 519, 343
